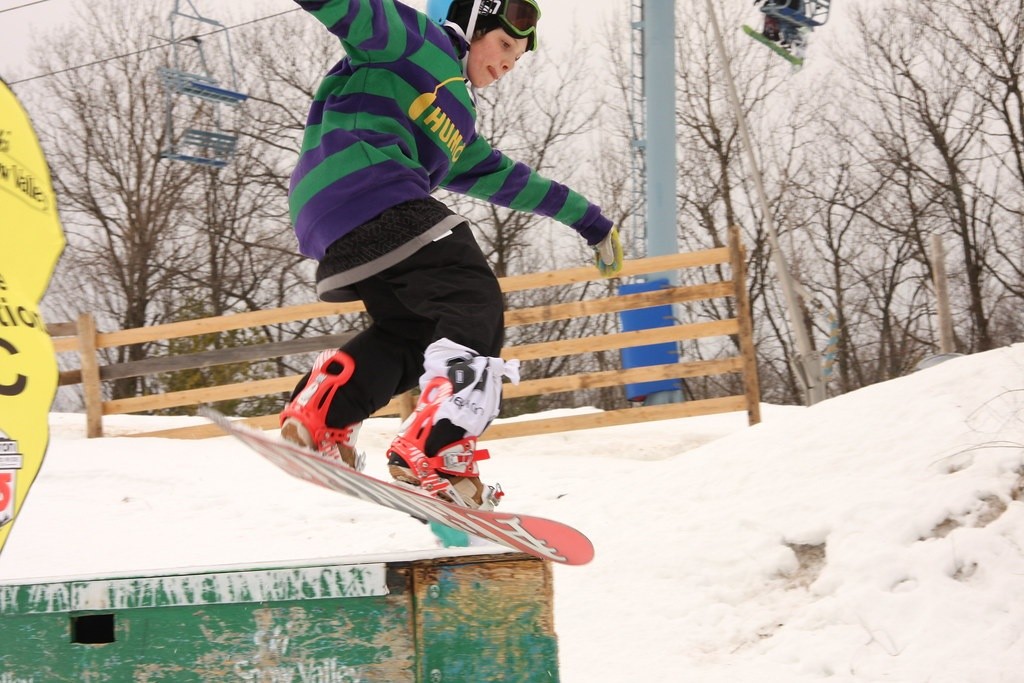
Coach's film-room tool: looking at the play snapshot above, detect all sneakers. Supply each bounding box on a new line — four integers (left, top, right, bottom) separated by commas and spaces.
281, 346, 367, 474
385, 376, 505, 512
780, 40, 801, 48
760, 29, 780, 41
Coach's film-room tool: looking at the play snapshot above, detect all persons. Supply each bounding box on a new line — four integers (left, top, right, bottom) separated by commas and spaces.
279, 1, 623, 512
753, 0, 807, 54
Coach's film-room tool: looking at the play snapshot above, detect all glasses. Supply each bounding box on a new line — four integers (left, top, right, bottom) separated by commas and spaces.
497, 0, 541, 52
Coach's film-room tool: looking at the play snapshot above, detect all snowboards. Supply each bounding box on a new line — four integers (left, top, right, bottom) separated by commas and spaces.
196, 405, 595, 569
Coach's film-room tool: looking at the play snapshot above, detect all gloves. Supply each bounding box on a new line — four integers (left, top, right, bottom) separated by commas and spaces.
590, 224, 623, 277
